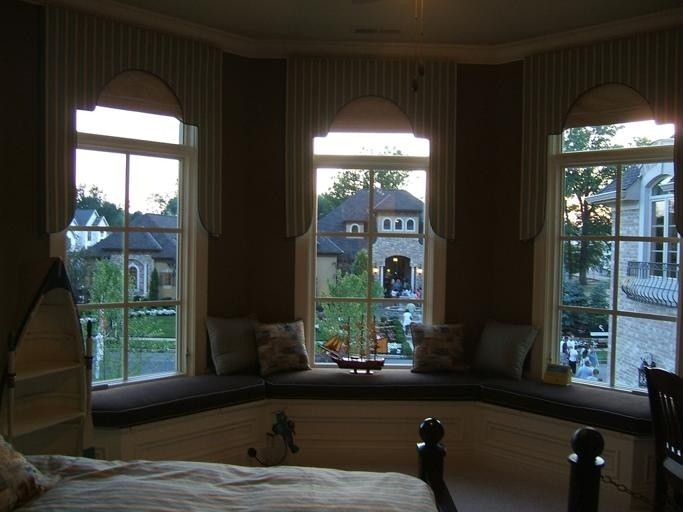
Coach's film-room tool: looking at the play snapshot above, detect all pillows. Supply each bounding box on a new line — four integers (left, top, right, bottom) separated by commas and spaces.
410, 323, 476, 374
256, 318, 316, 372
208, 312, 261, 374
0, 429, 47, 512
476, 324, 542, 382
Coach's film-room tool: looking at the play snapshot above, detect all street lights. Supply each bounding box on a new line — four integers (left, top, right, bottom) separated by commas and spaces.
373, 263, 381, 283
414, 265, 423, 294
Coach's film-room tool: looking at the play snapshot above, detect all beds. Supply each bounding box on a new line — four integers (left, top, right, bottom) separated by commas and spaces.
15, 420, 459, 512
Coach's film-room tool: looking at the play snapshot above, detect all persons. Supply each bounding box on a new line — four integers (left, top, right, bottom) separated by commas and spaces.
406, 301, 416, 317
559, 333, 602, 381
384, 268, 423, 310
402, 308, 413, 335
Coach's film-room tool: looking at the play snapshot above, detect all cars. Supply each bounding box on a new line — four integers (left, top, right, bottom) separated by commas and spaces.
131, 308, 176, 317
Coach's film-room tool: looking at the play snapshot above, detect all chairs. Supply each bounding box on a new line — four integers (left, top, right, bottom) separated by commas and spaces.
643, 365, 682, 512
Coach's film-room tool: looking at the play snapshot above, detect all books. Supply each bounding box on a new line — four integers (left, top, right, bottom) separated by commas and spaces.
458, 360, 489, 383
